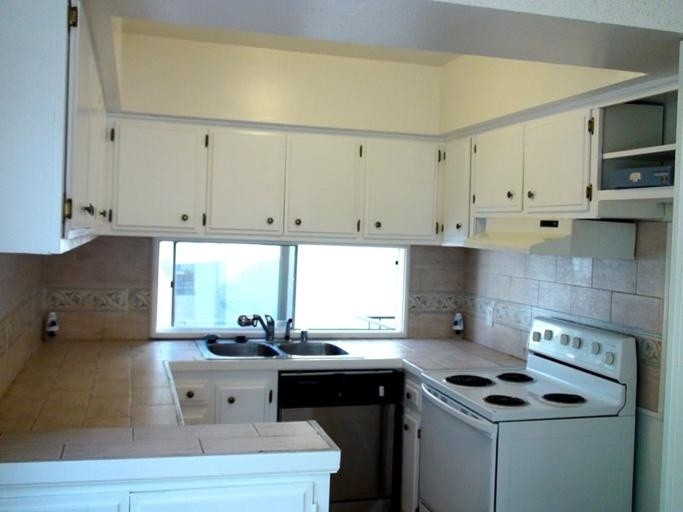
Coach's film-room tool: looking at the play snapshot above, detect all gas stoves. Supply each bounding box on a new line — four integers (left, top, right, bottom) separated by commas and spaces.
418, 353, 628, 424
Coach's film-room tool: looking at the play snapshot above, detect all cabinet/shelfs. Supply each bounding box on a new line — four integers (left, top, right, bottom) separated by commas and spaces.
112, 117, 201, 239
400, 379, 422, 512
62, 0, 108, 253
363, 135, 438, 246
473, 105, 665, 219
174, 378, 277, 427
440, 137, 471, 248
591, 82, 677, 201
0, 478, 321, 512
205, 124, 362, 246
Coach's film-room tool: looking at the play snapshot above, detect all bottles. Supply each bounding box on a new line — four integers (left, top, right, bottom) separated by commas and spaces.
451, 311, 464, 340
44, 311, 59, 342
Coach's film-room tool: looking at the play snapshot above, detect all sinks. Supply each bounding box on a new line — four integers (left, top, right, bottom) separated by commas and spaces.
266, 337, 361, 359
194, 336, 287, 358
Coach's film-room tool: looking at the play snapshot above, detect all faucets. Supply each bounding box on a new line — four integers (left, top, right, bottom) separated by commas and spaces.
253, 314, 275, 343
284, 318, 292, 339
